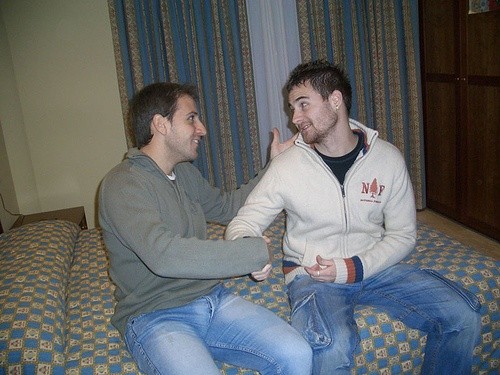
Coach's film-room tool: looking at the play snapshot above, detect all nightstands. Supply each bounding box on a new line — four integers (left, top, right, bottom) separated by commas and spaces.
8, 206, 88, 230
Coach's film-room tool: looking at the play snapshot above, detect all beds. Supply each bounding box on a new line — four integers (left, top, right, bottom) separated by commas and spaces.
1, 218, 499, 375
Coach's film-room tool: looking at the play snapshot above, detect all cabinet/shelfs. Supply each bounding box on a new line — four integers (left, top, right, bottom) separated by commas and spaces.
419, 0, 499, 240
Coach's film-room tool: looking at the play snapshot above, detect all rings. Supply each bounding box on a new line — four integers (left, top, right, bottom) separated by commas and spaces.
318, 270, 320, 277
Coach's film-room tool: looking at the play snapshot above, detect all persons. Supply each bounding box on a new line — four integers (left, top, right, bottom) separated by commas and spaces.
222, 59, 483, 375
97, 83, 314, 375
